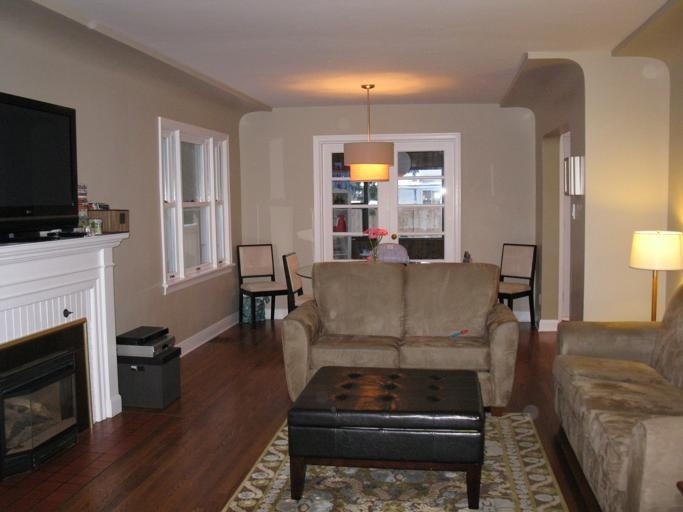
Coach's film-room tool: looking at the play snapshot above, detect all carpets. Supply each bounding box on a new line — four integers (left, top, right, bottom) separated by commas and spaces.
224, 413, 567, 512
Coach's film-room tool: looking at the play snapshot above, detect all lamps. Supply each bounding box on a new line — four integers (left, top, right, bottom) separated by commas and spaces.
342, 83, 394, 184
628, 229, 683, 322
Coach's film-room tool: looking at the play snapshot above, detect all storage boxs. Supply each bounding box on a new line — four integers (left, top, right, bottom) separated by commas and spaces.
87, 210, 129, 235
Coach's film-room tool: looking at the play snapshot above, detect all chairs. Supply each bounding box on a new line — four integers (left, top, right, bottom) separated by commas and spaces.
492, 242, 536, 332
237, 246, 313, 326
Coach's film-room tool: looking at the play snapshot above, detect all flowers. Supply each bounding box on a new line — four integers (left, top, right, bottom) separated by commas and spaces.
360, 228, 387, 259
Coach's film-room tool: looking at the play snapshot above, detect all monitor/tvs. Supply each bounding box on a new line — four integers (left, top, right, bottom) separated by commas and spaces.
0, 92, 79, 248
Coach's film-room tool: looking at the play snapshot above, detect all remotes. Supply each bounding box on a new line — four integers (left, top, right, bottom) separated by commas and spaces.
48, 232, 87, 240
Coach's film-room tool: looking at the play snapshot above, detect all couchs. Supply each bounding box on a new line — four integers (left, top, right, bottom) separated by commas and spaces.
554, 280, 683, 511
280, 258, 519, 412
